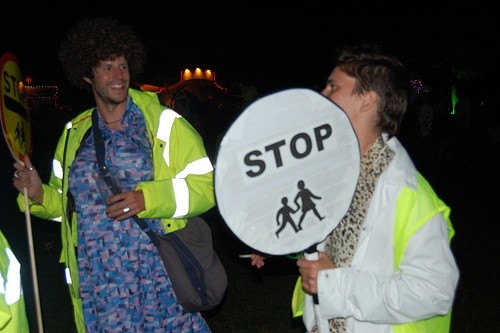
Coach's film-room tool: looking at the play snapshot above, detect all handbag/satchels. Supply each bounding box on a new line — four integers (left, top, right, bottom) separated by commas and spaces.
152, 216, 227, 312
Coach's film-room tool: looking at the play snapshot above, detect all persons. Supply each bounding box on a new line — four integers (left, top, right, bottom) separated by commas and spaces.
12, 18, 216, 333
251, 50, 460, 333
0, 231, 31, 333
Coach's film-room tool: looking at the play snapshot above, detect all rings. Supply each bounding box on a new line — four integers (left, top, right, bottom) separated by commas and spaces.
122, 207, 130, 214
14, 171, 19, 178
27, 166, 35, 171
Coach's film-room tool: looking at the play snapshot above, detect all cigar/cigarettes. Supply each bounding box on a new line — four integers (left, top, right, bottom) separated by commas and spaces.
240, 254, 252, 259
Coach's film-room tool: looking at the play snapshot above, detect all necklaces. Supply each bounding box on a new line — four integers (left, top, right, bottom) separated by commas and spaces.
105, 119, 120, 125
